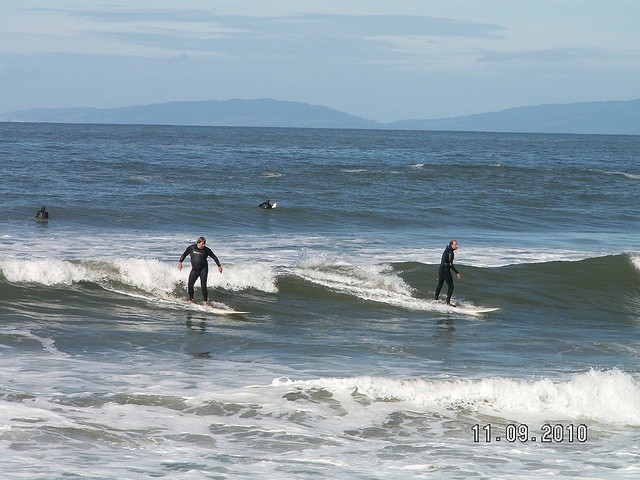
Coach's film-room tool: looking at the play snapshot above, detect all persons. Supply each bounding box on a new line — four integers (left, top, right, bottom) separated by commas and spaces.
434, 240, 461, 308
177, 236, 223, 308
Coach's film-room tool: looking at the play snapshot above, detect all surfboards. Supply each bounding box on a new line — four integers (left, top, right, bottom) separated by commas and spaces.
455, 305, 500, 313
190, 302, 251, 314
